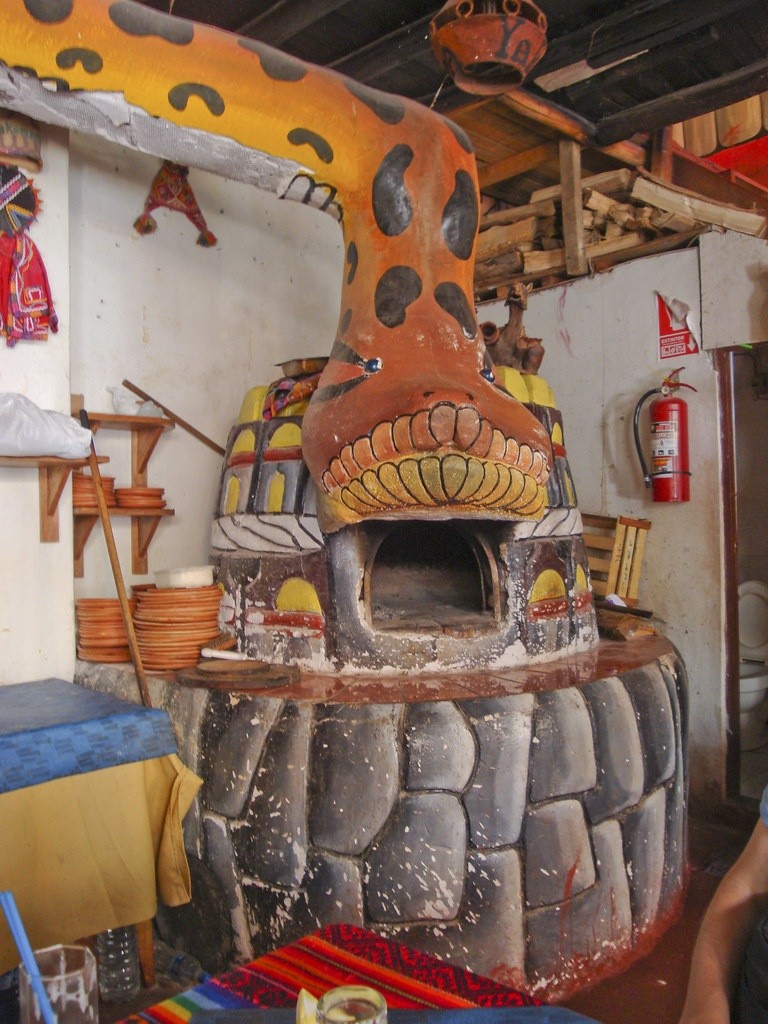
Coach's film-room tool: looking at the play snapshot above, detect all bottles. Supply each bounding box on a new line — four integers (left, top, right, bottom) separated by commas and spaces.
152, 938, 212, 985
89, 923, 141, 1004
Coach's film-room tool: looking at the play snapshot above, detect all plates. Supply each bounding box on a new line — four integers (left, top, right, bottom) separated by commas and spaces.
73, 472, 118, 508
129, 581, 224, 671
113, 487, 167, 508
74, 598, 140, 663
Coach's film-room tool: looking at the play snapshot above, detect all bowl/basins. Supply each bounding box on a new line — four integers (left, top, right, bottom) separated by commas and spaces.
273, 356, 330, 378
154, 566, 215, 588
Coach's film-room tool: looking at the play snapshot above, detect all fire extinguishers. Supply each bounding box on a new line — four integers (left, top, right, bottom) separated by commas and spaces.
631, 366, 697, 503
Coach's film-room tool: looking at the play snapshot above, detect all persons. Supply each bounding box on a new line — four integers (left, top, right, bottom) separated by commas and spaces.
676, 784, 768, 1024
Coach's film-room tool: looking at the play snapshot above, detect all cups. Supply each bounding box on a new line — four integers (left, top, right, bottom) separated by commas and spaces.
18, 944, 99, 1024
315, 984, 388, 1024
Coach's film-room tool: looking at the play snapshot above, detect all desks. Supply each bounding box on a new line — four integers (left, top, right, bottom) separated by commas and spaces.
102, 924, 539, 1024
0, 679, 204, 987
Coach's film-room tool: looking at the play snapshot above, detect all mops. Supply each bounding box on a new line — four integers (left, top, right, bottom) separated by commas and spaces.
86, 454, 238, 972
